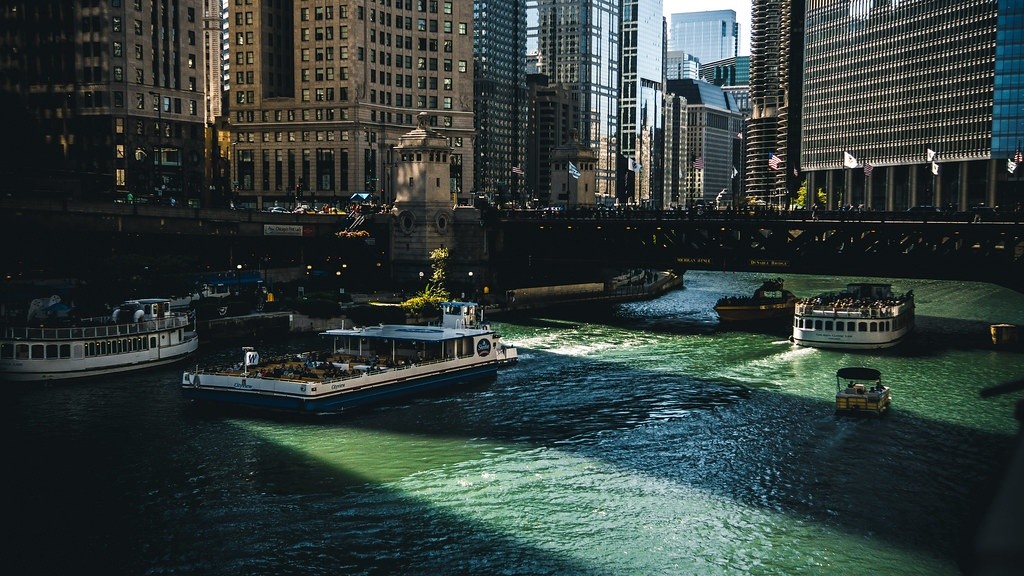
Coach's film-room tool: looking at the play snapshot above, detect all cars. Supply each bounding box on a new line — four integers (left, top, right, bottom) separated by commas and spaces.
319, 208, 346, 214
292, 205, 317, 214
907, 206, 932, 211
262, 207, 290, 213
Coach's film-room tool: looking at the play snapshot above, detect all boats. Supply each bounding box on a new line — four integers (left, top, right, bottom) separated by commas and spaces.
789, 282, 916, 350
180, 301, 519, 414
0, 298, 199, 381
714, 278, 799, 330
835, 367, 890, 415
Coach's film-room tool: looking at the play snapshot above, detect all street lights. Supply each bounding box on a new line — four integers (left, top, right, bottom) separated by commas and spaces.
237, 264, 242, 294
307, 266, 312, 297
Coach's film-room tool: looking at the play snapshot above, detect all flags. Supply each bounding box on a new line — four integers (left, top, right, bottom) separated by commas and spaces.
512, 167, 523, 174
927, 149, 935, 161
843, 152, 858, 168
769, 153, 781, 170
794, 169, 798, 176
693, 155, 704, 169
932, 162, 939, 175
569, 162, 581, 179
1014, 150, 1022, 164
1008, 159, 1017, 173
731, 167, 738, 178
628, 158, 641, 173
864, 163, 873, 176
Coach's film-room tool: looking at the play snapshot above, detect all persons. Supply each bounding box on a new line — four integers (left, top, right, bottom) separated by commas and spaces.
804, 293, 904, 309
196, 349, 423, 379
323, 202, 390, 213
717, 295, 751, 305
128, 193, 133, 205
848, 380, 885, 394
